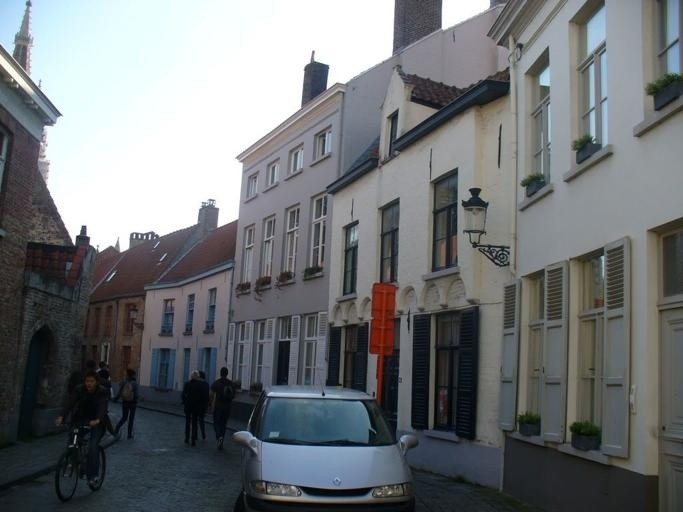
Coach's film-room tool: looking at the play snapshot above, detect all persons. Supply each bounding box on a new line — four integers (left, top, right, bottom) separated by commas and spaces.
55, 361, 122, 481
111, 368, 139, 440
209, 367, 236, 450
181, 370, 209, 445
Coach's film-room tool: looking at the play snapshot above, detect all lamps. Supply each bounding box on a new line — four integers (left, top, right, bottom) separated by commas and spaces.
461, 187, 489, 248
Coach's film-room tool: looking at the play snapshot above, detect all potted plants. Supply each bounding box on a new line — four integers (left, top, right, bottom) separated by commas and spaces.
569, 135, 600, 163
570, 419, 602, 452
521, 171, 545, 197
513, 410, 540, 436
645, 72, 683, 111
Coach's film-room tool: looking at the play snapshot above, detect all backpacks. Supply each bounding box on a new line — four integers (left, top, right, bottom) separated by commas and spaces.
121, 381, 135, 401
224, 385, 233, 399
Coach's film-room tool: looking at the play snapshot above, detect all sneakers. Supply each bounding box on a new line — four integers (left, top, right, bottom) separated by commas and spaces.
184, 435, 208, 446
216, 436, 224, 450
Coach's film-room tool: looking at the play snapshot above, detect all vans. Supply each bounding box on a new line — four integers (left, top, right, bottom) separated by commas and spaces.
230, 383, 421, 510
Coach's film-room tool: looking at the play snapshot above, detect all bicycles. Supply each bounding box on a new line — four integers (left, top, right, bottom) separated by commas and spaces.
53, 420, 107, 504
